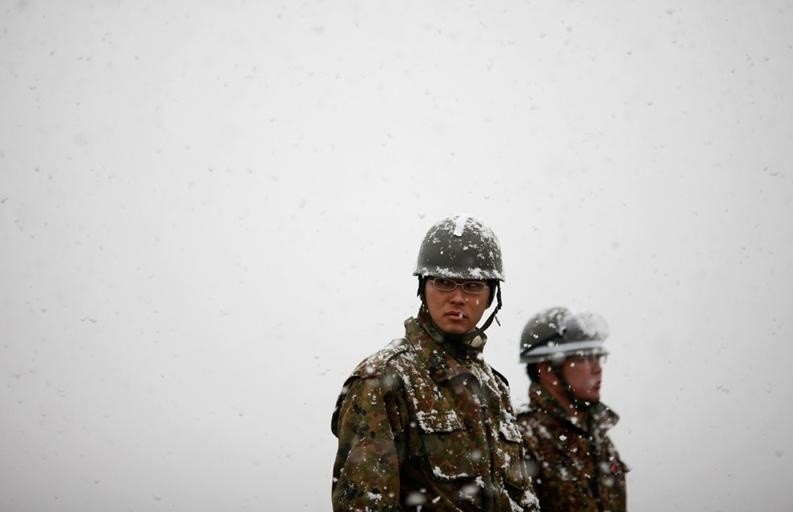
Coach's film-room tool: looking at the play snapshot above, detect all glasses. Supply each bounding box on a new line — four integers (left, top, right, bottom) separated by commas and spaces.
427, 275, 489, 294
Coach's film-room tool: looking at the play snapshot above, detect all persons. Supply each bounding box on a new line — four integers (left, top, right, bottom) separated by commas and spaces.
332, 215, 541, 512
514, 305, 630, 512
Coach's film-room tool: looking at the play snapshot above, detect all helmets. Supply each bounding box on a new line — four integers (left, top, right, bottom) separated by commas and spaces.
413, 215, 505, 283
520, 307, 610, 363
